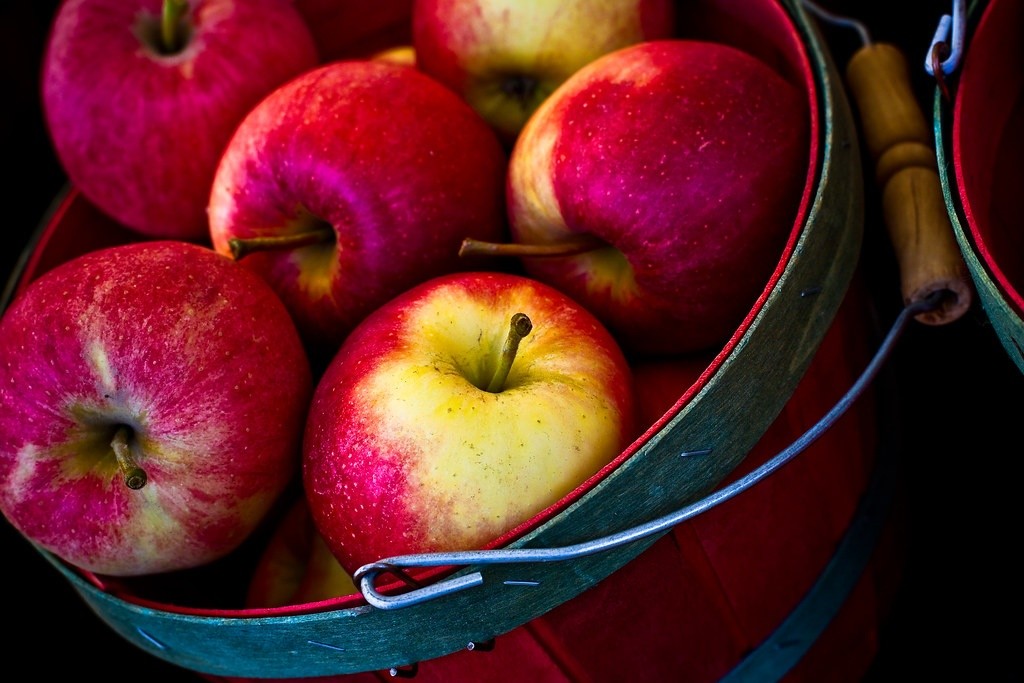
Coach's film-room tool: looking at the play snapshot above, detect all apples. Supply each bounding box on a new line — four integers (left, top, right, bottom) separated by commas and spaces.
0, 0, 812, 612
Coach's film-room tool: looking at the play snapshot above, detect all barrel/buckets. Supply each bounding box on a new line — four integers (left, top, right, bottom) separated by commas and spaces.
2, 0, 974, 683
925, 0, 1024, 378
